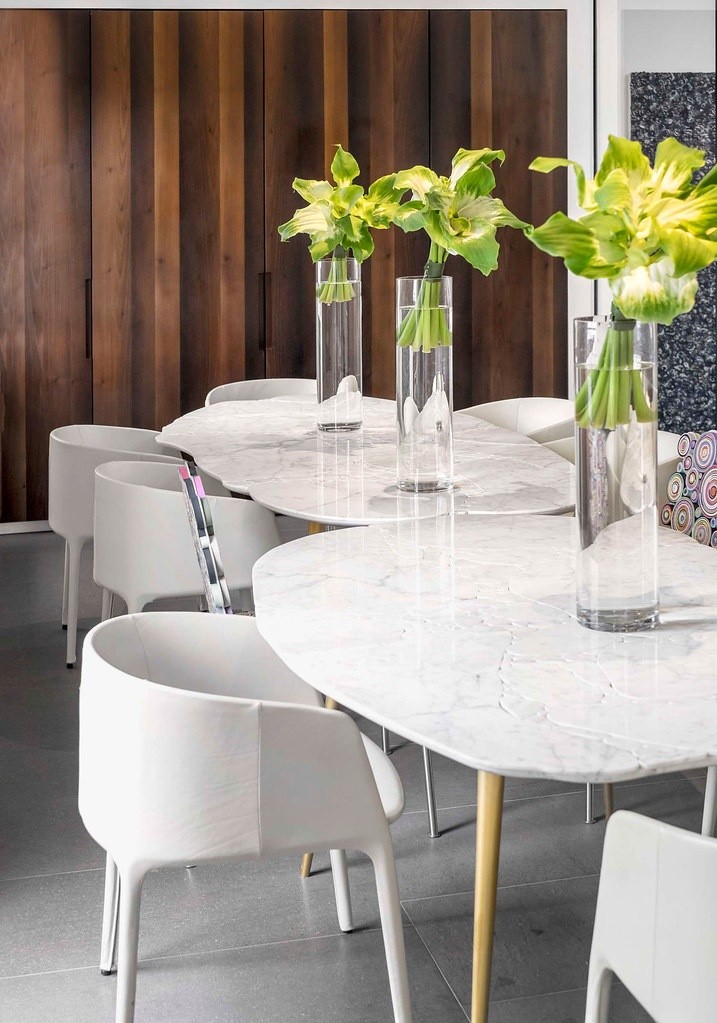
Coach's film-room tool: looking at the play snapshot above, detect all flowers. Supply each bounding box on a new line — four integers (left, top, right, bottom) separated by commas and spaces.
387, 146, 533, 279
276, 142, 406, 262
523, 129, 717, 324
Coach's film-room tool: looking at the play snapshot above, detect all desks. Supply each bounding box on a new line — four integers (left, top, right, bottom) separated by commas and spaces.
251, 514, 716, 1023
154, 393, 578, 537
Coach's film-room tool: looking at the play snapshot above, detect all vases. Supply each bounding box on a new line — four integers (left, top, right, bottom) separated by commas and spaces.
573, 314, 661, 631
313, 259, 362, 432
394, 275, 455, 494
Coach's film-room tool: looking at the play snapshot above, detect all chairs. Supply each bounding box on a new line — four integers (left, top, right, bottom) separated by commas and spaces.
78, 611, 417, 1021
92, 458, 281, 628
48, 425, 197, 671
205, 376, 316, 410
580, 811, 717, 1023
542, 424, 691, 509
